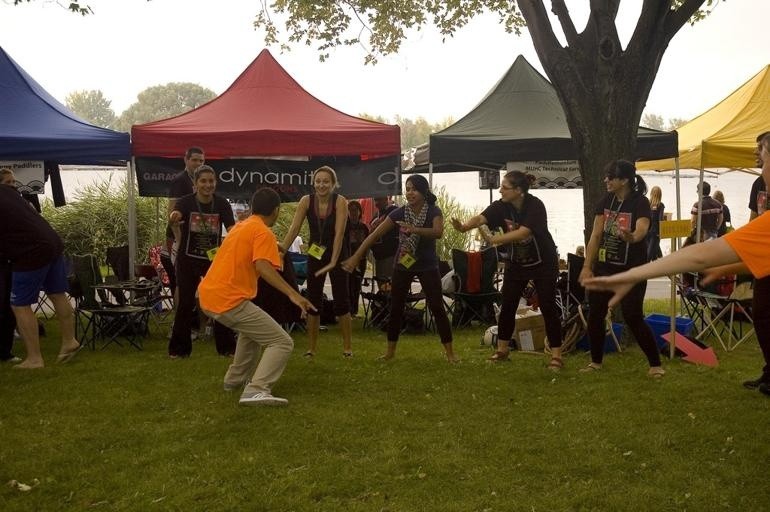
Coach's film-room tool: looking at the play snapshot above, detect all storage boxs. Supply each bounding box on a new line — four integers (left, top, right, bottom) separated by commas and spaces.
576, 321, 624, 356
645, 311, 695, 354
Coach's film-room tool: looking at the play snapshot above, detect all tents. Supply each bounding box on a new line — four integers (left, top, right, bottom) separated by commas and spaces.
126, 49, 400, 319
630, 63, 769, 360
398, 53, 678, 362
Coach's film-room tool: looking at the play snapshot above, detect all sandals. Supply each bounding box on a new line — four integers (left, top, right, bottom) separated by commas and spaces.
487, 352, 509, 361
547, 357, 563, 368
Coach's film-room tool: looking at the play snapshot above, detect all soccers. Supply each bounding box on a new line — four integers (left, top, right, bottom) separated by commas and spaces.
484, 326, 498, 346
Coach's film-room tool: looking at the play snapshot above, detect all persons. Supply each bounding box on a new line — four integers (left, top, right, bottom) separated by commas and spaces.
165, 147, 237, 359
450, 170, 565, 370
576, 133, 770, 398
1, 168, 23, 363
338, 175, 456, 362
199, 186, 316, 405
1, 183, 84, 370
269, 165, 400, 357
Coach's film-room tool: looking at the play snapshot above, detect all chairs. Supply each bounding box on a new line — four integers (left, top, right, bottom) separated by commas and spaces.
281, 247, 587, 335
666, 225, 755, 352
34, 245, 172, 355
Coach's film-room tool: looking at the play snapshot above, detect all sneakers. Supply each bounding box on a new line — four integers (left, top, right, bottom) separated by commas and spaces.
743, 372, 770, 396
239, 391, 289, 407
646, 367, 665, 378
579, 363, 603, 373
224, 382, 242, 392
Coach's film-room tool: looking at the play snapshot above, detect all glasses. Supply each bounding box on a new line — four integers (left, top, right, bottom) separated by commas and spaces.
606, 174, 619, 180
500, 184, 516, 190
